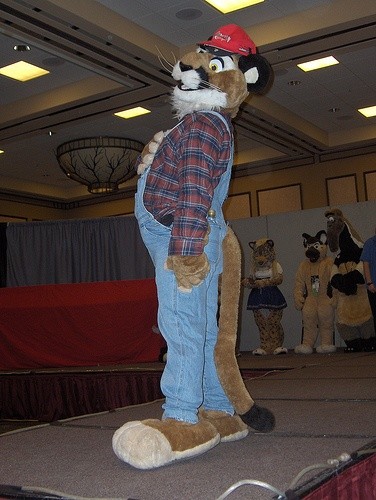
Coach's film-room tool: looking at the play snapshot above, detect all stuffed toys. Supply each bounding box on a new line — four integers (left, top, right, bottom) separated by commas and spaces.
324, 208, 376, 353
113, 23, 274, 463
293, 230, 338, 354
241, 238, 288, 355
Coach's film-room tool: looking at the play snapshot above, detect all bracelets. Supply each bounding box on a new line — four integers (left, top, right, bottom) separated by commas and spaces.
367, 282, 373, 286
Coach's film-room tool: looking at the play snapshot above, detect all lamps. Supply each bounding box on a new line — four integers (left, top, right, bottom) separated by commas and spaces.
56, 136, 145, 194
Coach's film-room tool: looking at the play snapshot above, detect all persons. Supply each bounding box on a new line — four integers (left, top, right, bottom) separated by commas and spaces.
360, 235, 376, 333
